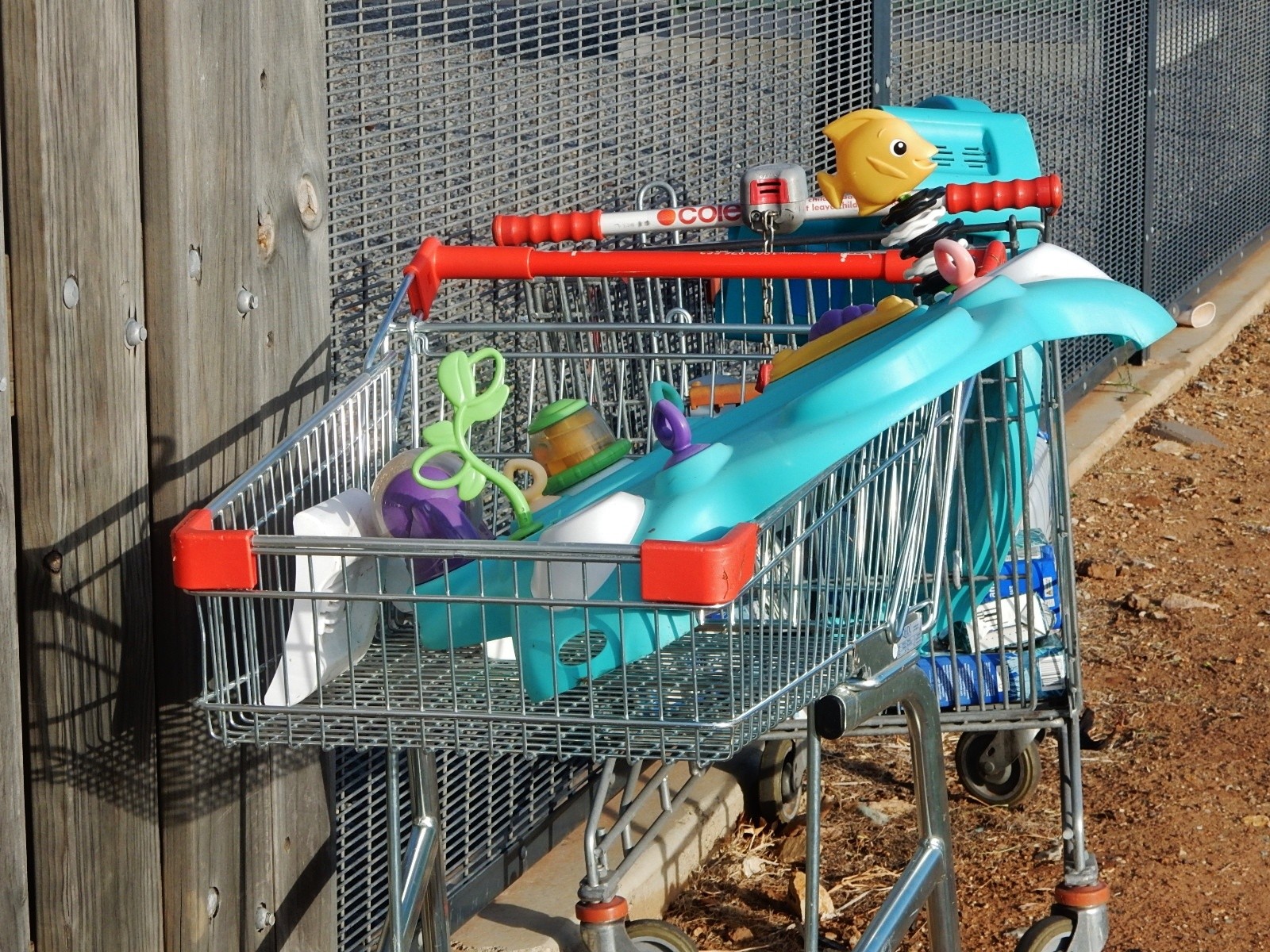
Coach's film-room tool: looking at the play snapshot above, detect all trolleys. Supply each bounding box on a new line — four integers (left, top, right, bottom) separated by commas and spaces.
169, 162, 1112, 952
